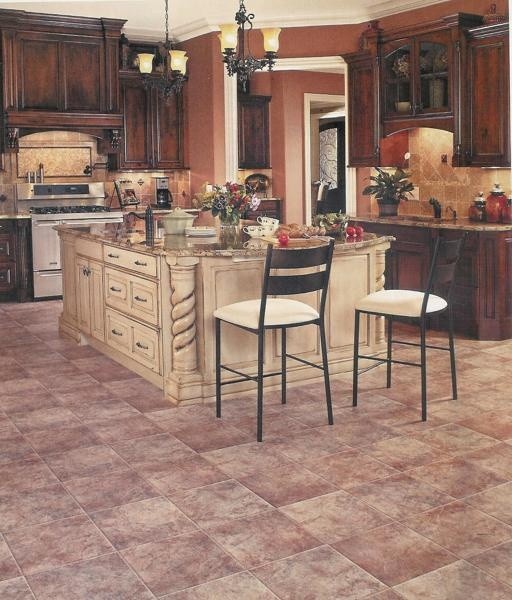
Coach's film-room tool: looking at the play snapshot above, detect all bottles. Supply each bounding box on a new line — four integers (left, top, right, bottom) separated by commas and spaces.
145, 203, 154, 246
468, 182, 512, 225
27, 163, 44, 183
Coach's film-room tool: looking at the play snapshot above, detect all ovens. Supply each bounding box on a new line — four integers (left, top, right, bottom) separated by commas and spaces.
31, 218, 123, 298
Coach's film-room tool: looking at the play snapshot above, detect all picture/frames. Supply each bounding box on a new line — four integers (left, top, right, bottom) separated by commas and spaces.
113, 178, 140, 206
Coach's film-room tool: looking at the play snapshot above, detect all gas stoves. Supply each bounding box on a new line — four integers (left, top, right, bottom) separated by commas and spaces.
15, 182, 124, 221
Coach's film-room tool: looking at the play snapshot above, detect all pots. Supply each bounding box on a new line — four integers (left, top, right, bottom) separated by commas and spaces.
159, 207, 199, 234
163, 234, 188, 249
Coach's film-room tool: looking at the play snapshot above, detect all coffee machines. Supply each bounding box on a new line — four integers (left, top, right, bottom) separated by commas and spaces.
151, 177, 173, 210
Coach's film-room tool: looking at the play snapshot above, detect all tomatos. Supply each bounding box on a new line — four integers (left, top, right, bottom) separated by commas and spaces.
279, 234, 289, 244
347, 226, 355, 235
356, 235, 362, 242
355, 227, 363, 235
347, 236, 354, 242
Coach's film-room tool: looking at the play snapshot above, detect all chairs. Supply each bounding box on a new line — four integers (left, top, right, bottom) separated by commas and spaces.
353, 232, 468, 423
213, 239, 335, 442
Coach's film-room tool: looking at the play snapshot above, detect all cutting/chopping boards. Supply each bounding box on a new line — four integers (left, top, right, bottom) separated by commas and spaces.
262, 238, 312, 243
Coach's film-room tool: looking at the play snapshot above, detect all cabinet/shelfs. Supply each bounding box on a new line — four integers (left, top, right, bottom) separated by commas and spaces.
0, 219, 17, 295
0, 8, 128, 129
101, 243, 161, 377
52, 233, 76, 326
238, 95, 272, 169
366, 224, 511, 341
242, 198, 282, 223
72, 235, 106, 344
32, 218, 63, 300
108, 70, 190, 171
339, 11, 511, 167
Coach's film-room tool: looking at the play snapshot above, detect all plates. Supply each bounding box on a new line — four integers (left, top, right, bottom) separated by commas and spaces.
186, 238, 217, 243
184, 226, 216, 236
245, 173, 270, 192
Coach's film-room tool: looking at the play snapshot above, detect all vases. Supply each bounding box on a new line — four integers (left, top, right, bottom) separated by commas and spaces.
216, 223, 239, 250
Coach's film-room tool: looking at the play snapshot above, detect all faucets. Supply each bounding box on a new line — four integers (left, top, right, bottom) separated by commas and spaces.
430, 198, 441, 218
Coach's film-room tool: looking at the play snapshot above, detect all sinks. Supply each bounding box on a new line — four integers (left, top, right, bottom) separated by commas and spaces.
407, 216, 461, 225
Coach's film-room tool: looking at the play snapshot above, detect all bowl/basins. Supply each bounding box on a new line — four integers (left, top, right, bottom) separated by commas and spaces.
394, 102, 410, 113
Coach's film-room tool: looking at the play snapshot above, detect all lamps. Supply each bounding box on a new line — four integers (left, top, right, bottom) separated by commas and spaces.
217, 1, 282, 92
136, 0, 190, 105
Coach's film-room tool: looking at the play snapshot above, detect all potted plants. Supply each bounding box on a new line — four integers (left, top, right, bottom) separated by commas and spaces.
362, 166, 413, 217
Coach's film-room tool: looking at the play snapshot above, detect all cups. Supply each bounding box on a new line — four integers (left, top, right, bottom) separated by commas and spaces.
242, 239, 270, 249
243, 216, 280, 238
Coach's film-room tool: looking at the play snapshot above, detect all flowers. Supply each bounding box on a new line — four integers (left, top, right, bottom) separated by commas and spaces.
193, 181, 261, 224
